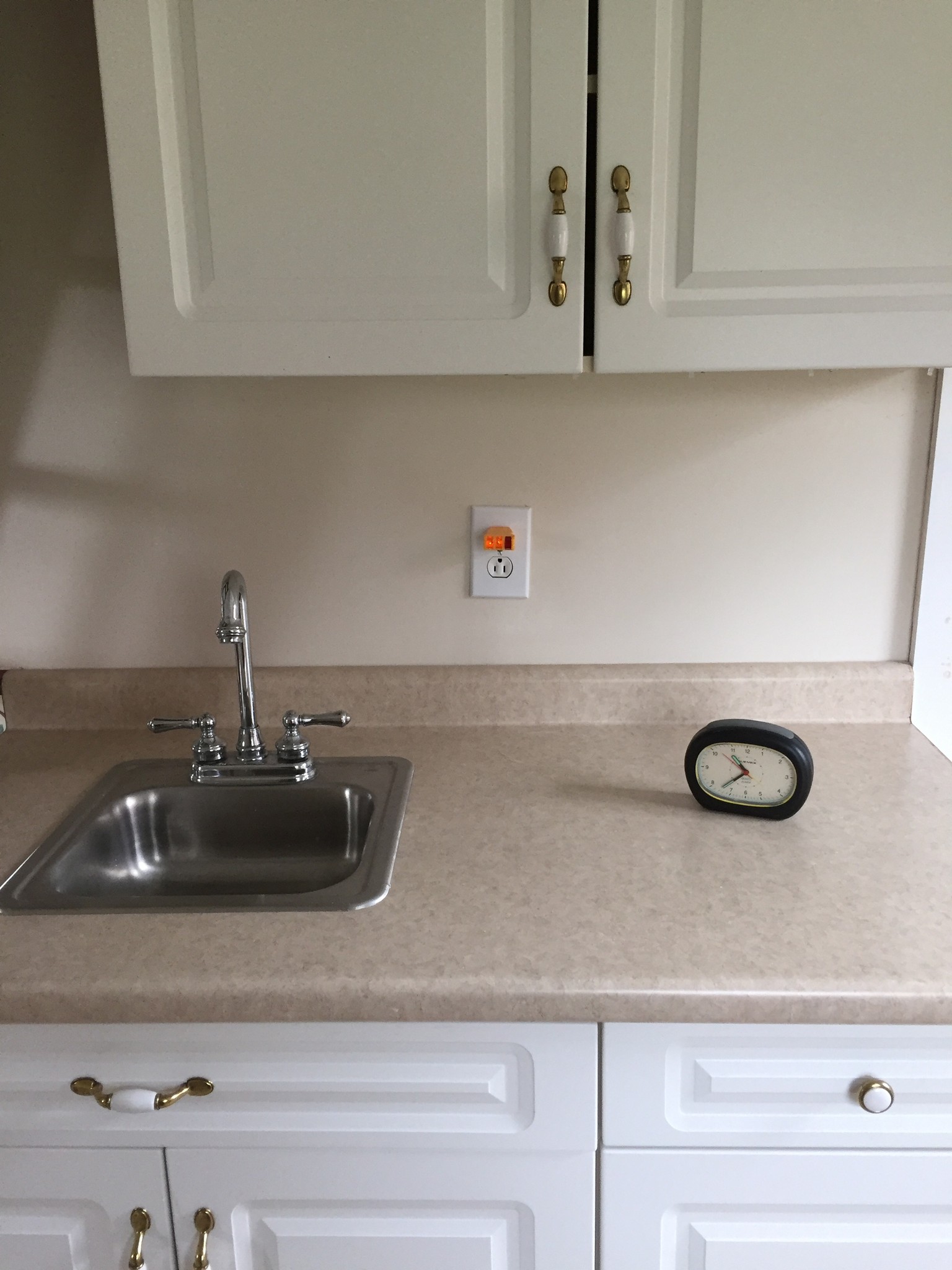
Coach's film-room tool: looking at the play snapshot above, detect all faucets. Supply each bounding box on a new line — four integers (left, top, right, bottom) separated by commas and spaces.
144, 567, 352, 784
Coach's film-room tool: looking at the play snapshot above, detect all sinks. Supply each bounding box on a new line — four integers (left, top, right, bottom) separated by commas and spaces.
1, 755, 413, 915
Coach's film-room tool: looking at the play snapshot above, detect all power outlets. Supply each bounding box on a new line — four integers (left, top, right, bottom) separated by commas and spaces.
469, 506, 532, 597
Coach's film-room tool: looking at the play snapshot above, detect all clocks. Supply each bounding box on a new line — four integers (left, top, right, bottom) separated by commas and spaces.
684, 718, 814, 821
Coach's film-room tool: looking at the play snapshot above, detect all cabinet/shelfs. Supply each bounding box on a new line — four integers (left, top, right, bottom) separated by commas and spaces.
0, 1022, 598, 1270
93, 0, 952, 374
598, 1021, 952, 1270
